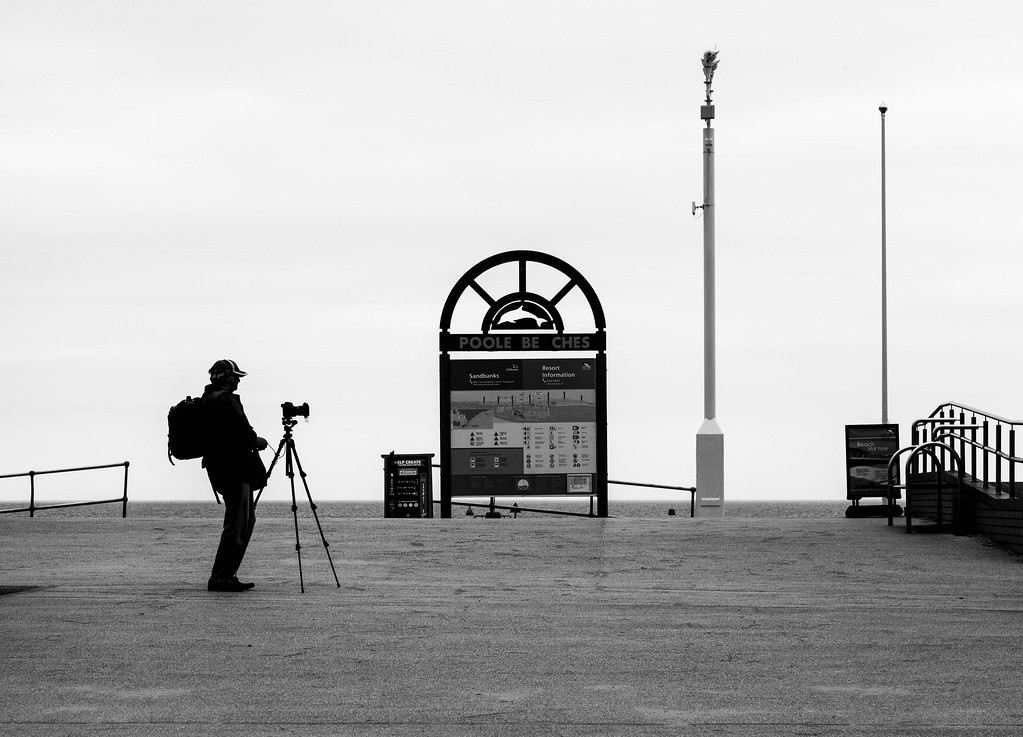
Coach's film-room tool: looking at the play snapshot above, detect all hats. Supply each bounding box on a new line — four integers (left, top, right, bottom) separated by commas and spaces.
209, 360, 248, 377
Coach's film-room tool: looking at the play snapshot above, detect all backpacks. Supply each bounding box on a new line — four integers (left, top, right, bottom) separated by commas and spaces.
167, 390, 233, 466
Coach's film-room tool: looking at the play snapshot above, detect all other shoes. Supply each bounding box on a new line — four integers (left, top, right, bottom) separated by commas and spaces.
208, 575, 255, 592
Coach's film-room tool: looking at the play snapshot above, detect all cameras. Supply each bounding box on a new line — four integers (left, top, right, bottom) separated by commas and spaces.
281, 402, 309, 419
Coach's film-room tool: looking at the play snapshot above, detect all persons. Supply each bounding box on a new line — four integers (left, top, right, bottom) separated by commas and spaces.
202, 359, 268, 593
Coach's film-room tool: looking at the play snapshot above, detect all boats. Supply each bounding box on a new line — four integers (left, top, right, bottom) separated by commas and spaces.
668, 507, 675, 515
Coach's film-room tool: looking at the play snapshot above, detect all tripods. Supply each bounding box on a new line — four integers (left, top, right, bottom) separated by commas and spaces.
255, 418, 340, 593
